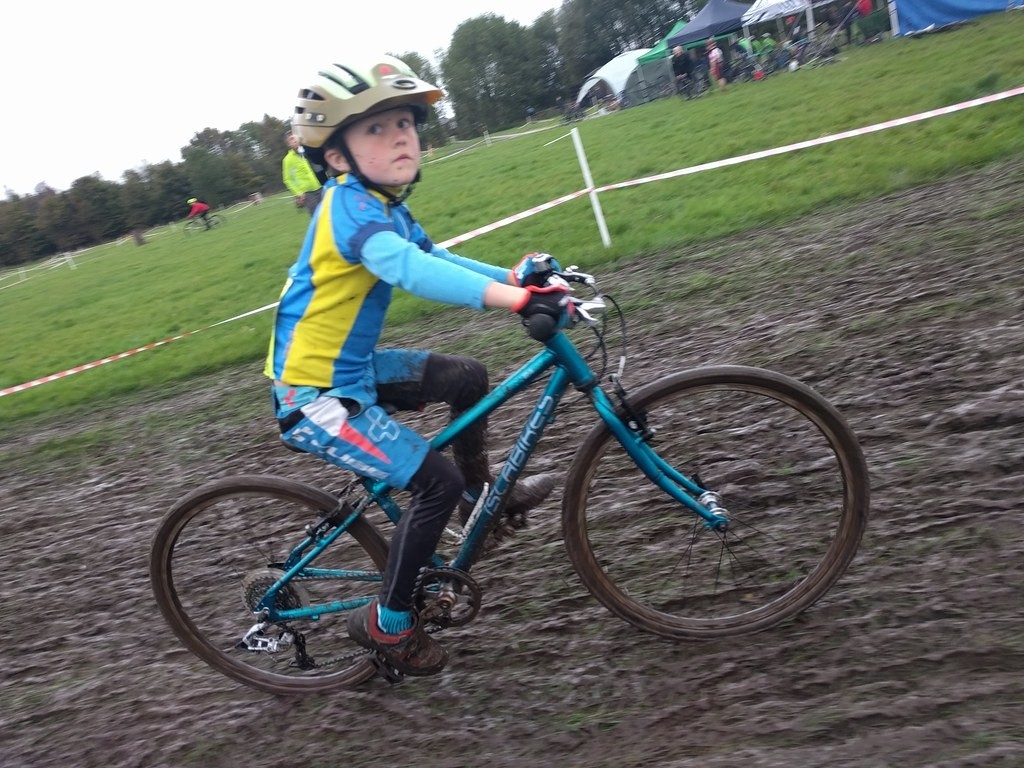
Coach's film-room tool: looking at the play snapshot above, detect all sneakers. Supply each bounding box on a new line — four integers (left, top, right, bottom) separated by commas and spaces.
460, 471, 551, 530
349, 597, 450, 674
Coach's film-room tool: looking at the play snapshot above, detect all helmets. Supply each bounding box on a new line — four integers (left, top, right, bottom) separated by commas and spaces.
187, 198, 197, 206
291, 53, 443, 155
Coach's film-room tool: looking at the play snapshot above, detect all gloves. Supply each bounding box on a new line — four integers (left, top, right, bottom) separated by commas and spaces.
512, 252, 562, 290
516, 284, 575, 324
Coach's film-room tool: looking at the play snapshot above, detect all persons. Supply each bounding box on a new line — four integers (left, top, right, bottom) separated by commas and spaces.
262, 53, 573, 678
554, 92, 565, 109
521, 102, 537, 123
185, 197, 212, 231
671, 0, 883, 102
281, 129, 325, 218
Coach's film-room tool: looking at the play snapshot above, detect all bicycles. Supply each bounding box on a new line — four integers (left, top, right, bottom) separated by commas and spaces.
183, 210, 227, 238
149, 251, 871, 700
